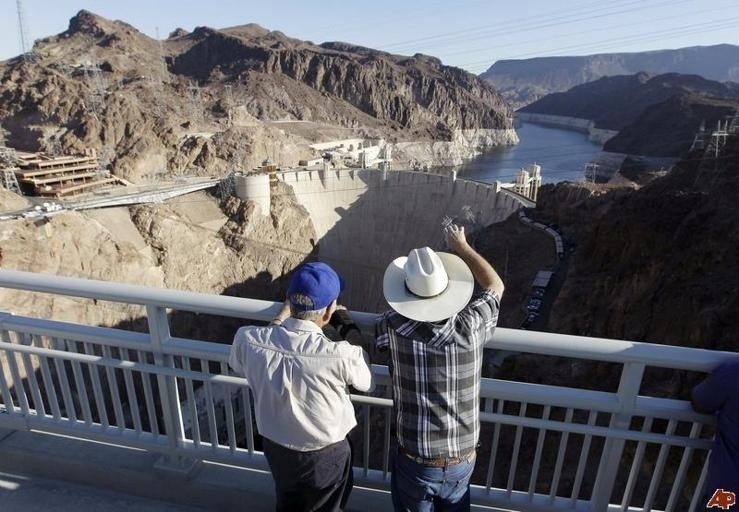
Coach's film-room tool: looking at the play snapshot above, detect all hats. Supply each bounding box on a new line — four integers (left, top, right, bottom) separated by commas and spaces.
382, 246, 475, 324
285, 259, 346, 313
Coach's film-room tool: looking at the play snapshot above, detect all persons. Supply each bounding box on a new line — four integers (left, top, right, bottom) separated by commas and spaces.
226, 262, 376, 512
377, 222, 507, 512
687, 354, 738, 511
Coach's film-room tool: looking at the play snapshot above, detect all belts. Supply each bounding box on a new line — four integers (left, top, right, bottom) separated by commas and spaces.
398, 448, 475, 467
